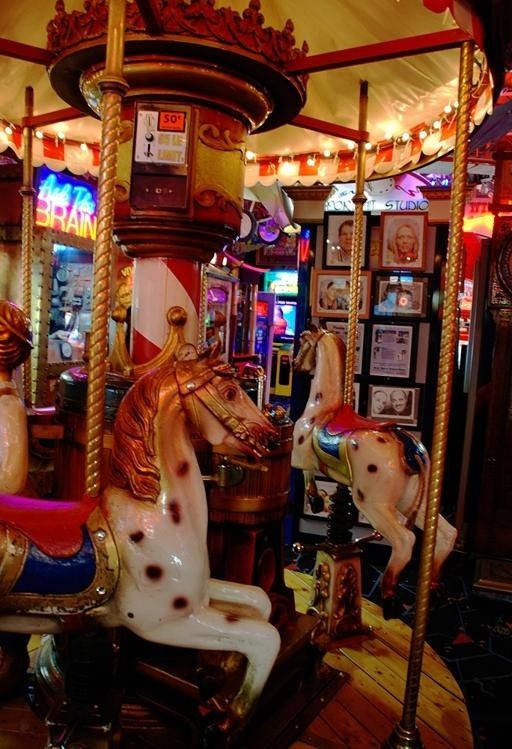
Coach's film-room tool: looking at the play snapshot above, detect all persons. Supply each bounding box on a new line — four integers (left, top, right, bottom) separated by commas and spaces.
329, 220, 355, 265
378, 388, 414, 416
374, 278, 404, 316
339, 280, 363, 310
374, 390, 389, 415
392, 222, 421, 266
395, 290, 414, 311
320, 282, 343, 311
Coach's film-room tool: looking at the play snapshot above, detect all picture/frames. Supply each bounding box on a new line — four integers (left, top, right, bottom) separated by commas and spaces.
310, 209, 431, 428
